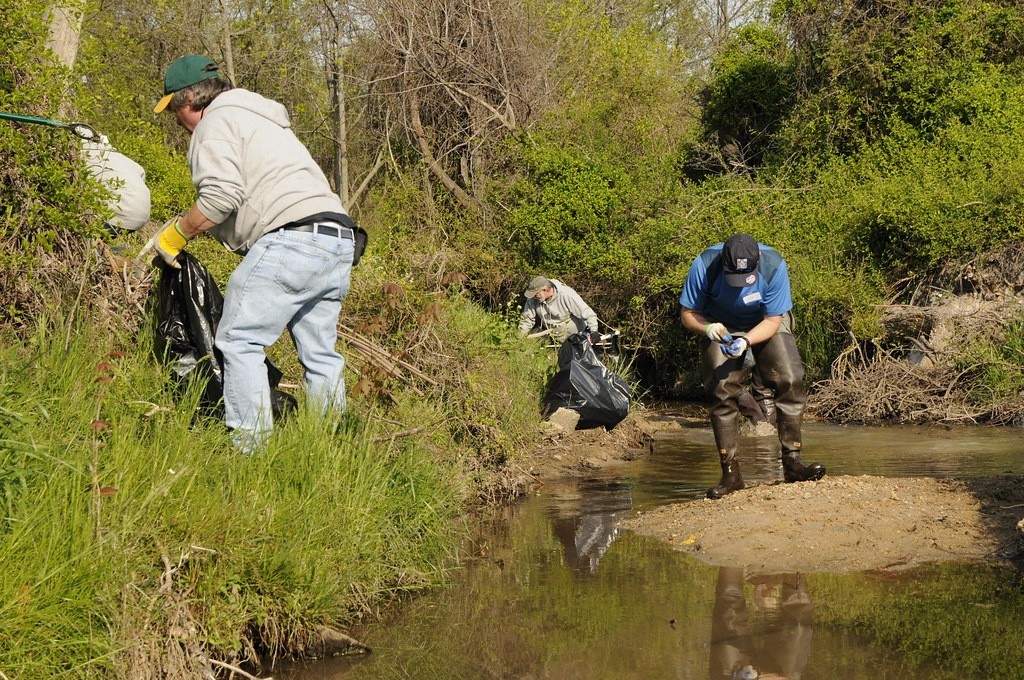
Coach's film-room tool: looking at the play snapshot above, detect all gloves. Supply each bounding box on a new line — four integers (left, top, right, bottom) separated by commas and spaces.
590, 332, 600, 346
152, 214, 196, 270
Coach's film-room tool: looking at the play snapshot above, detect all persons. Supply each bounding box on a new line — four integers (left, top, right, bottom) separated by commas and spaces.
679, 233, 826, 500
517, 275, 601, 355
152, 52, 357, 455
73, 120, 153, 242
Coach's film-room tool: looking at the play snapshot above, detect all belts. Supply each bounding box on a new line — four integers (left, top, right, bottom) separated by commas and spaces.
272, 223, 353, 239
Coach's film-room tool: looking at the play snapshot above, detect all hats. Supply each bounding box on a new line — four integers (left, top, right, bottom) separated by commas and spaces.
722, 234, 759, 287
153, 54, 219, 114
524, 276, 549, 298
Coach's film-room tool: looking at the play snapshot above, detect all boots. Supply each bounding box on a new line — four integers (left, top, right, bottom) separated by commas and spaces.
699, 335, 744, 497
751, 331, 824, 480
